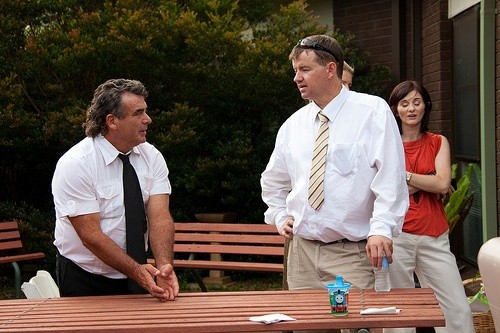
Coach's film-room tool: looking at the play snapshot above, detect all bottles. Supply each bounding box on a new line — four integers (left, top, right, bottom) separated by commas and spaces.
373, 251, 391, 292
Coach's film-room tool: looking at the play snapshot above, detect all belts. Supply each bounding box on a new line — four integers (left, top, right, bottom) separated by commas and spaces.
309, 238, 367, 246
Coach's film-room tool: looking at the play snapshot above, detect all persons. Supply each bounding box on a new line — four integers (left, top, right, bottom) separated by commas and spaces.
342, 60, 436, 333
383, 80, 475, 333
260, 34, 410, 333
51, 78, 179, 302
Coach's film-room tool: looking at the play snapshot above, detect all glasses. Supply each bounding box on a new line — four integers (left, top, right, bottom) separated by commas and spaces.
295, 38, 340, 63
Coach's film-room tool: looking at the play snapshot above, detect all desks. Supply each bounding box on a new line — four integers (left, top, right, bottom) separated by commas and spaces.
0, 289, 445, 333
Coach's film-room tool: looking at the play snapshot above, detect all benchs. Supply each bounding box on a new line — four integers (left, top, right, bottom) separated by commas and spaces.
147, 223, 286, 288
0, 221, 45, 299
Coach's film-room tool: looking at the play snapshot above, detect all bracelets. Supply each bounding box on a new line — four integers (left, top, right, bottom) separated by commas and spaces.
407, 172, 412, 181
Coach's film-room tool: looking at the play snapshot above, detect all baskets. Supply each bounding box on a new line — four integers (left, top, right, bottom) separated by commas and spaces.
471, 313, 496, 333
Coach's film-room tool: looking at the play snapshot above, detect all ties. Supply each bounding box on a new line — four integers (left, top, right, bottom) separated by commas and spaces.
117, 151, 147, 282
308, 113, 329, 209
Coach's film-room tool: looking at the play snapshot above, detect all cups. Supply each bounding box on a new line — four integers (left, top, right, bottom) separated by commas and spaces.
325, 276, 351, 316
